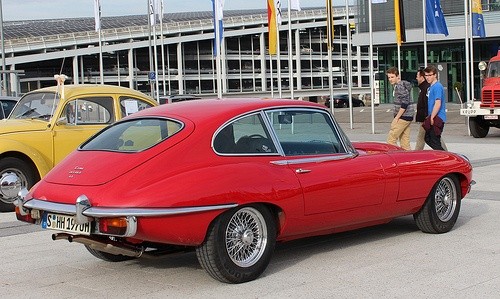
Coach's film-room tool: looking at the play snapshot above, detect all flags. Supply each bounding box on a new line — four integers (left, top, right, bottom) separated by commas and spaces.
325, 0, 336, 50
146, 0, 165, 27
393, 0, 407, 44
468, 0, 486, 38
265, 0, 302, 55
93, 0, 103, 31
421, 0, 449, 37
212, 0, 226, 56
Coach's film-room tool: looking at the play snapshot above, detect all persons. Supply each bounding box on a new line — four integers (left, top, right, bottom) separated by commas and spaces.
385, 67, 415, 151
415, 66, 449, 151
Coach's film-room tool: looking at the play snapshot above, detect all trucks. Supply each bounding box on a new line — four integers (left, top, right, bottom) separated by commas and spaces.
455, 50, 500, 138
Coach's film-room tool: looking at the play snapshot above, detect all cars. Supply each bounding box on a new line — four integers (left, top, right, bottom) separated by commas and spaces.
13, 97, 476, 284
0, 74, 181, 213
0, 94, 82, 122
285, 45, 314, 55
86, 65, 141, 75
325, 94, 365, 109
138, 95, 202, 111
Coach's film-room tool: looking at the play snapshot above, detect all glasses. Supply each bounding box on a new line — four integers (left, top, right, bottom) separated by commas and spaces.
424, 74, 434, 77
